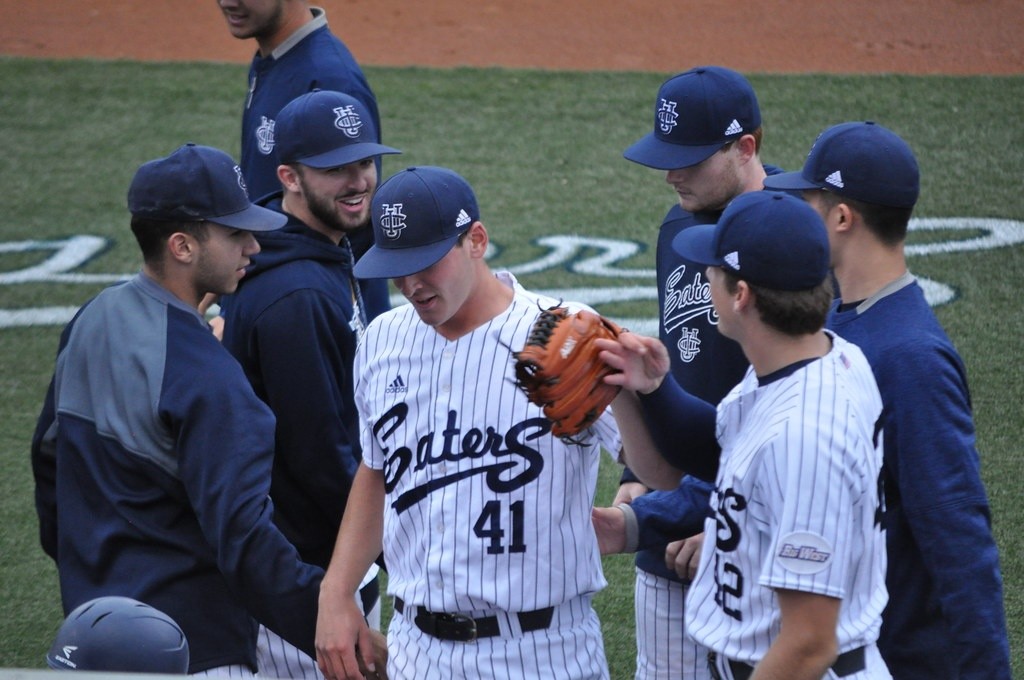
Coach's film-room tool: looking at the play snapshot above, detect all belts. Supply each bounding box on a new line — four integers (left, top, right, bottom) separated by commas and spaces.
707, 646, 866, 680
391, 597, 554, 643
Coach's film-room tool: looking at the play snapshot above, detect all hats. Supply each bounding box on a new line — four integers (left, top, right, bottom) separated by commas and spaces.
125, 143, 289, 232
274, 88, 404, 170
671, 190, 831, 292
622, 64, 761, 171
353, 167, 479, 280
763, 118, 920, 210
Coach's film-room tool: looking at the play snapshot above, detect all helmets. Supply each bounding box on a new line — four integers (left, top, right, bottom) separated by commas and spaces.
44, 596, 190, 676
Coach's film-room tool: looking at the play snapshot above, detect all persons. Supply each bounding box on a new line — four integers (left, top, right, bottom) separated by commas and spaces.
196, 0, 384, 344
591, 191, 898, 679
582, 121, 1018, 679
34, 146, 393, 678
613, 66, 841, 680
220, 87, 393, 678
318, 166, 686, 680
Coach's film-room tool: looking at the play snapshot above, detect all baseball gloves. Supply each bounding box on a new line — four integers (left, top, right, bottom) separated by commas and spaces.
492, 298, 632, 447
